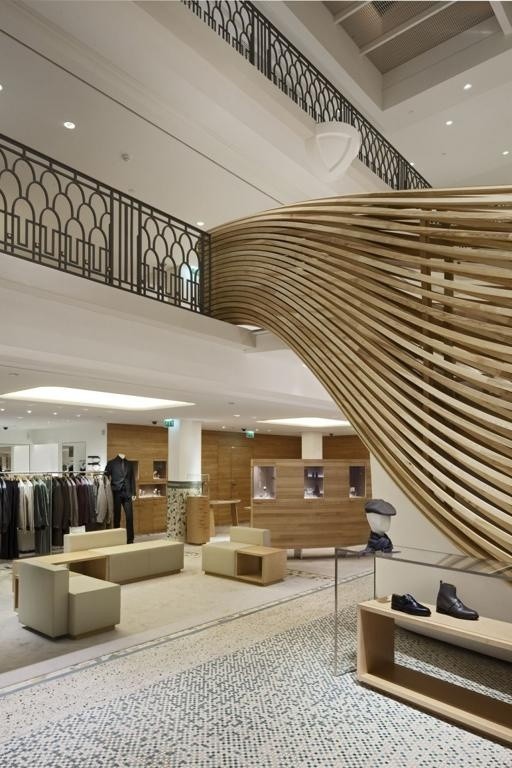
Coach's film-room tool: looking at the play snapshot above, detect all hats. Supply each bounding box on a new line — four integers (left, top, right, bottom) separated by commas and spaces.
364, 499, 397, 516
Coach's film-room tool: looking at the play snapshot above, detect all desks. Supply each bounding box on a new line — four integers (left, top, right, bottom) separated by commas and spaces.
328, 541, 511, 679
209, 498, 242, 527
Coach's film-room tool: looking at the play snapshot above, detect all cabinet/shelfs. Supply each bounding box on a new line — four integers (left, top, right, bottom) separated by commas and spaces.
354, 594, 511, 746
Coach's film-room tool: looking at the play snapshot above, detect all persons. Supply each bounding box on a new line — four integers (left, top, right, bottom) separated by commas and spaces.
103, 453, 137, 544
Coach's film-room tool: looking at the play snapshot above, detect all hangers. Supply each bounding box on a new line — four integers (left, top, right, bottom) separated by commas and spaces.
0, 470, 113, 490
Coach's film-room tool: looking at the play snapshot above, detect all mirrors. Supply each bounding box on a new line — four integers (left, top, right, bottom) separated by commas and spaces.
58, 440, 88, 477
1, 443, 31, 475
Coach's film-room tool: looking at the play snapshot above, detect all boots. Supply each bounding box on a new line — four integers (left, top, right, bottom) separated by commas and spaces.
436, 579, 480, 621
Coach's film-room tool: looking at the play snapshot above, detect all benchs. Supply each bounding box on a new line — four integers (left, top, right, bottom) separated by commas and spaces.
11, 526, 288, 641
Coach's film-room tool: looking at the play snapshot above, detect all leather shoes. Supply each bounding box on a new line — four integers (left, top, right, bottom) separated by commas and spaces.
391, 592, 432, 618
